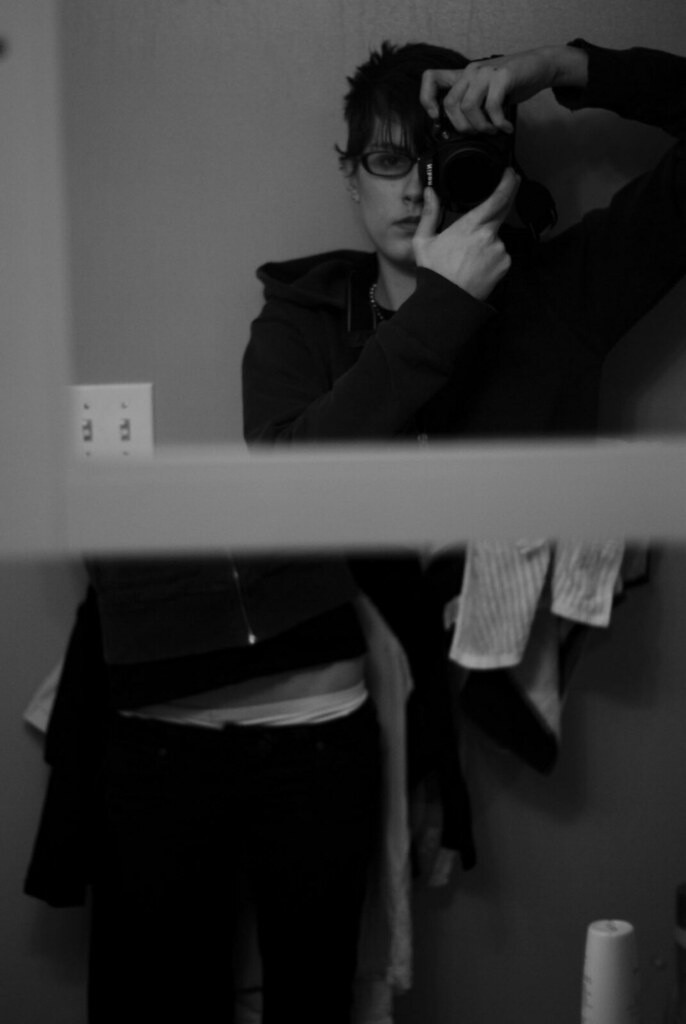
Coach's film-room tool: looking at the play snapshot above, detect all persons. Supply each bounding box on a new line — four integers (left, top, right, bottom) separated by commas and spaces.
24, 38, 685, 1023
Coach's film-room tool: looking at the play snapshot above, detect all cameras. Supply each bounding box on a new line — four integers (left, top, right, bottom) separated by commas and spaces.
412, 108, 516, 237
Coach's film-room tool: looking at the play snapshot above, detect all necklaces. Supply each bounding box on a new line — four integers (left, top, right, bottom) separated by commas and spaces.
370, 284, 384, 321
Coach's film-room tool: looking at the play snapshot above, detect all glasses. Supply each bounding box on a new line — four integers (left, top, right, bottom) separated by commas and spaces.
346, 151, 418, 179
